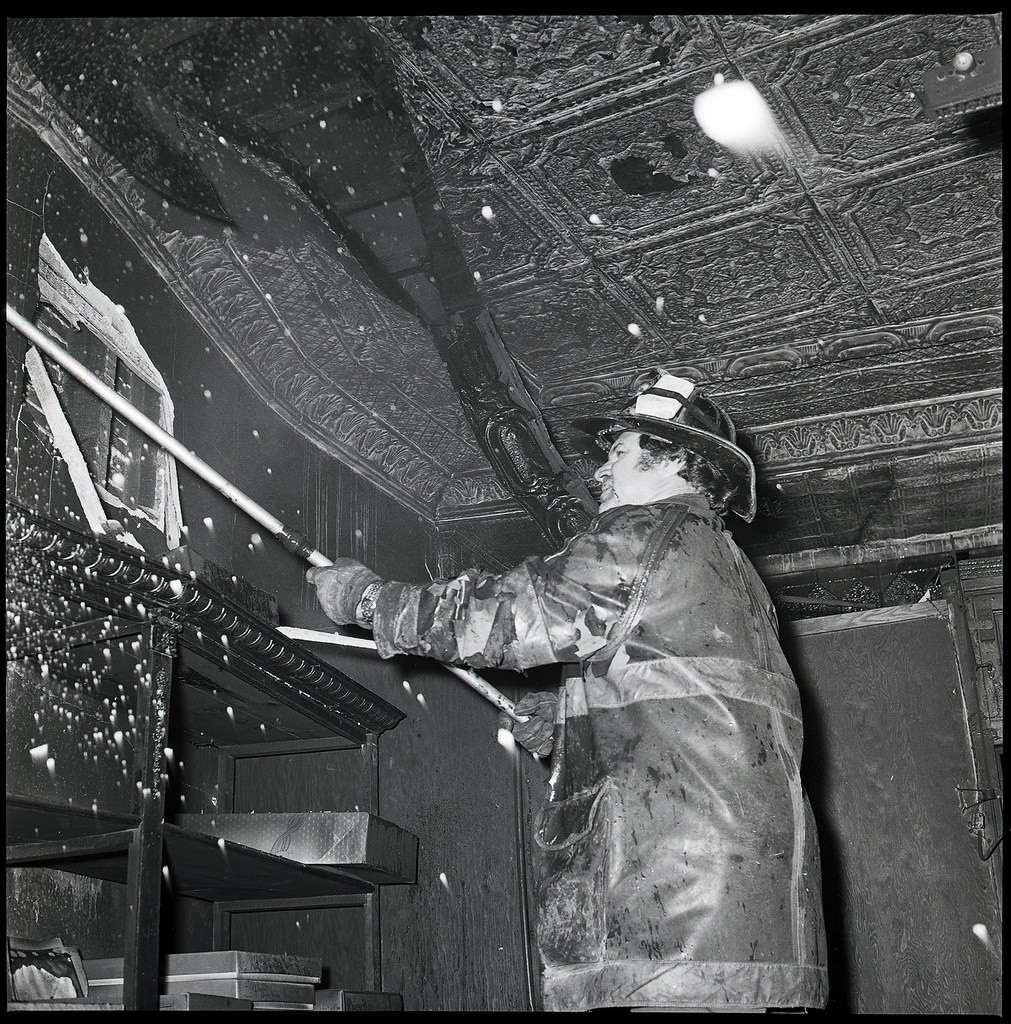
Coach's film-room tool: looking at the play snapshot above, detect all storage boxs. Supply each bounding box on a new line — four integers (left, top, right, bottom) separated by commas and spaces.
155, 545, 282, 630
184, 814, 418, 883
83, 950, 404, 1012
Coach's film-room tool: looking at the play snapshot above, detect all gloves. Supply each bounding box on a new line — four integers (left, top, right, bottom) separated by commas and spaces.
305, 555, 384, 630
495, 692, 561, 758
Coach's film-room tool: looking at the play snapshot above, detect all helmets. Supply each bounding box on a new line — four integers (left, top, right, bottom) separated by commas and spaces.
571, 367, 756, 524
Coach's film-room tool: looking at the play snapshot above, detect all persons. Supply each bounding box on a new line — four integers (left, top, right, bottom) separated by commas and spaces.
305, 376, 830, 1013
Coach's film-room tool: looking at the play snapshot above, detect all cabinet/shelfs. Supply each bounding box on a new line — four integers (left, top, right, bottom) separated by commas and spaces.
5, 503, 405, 1012
